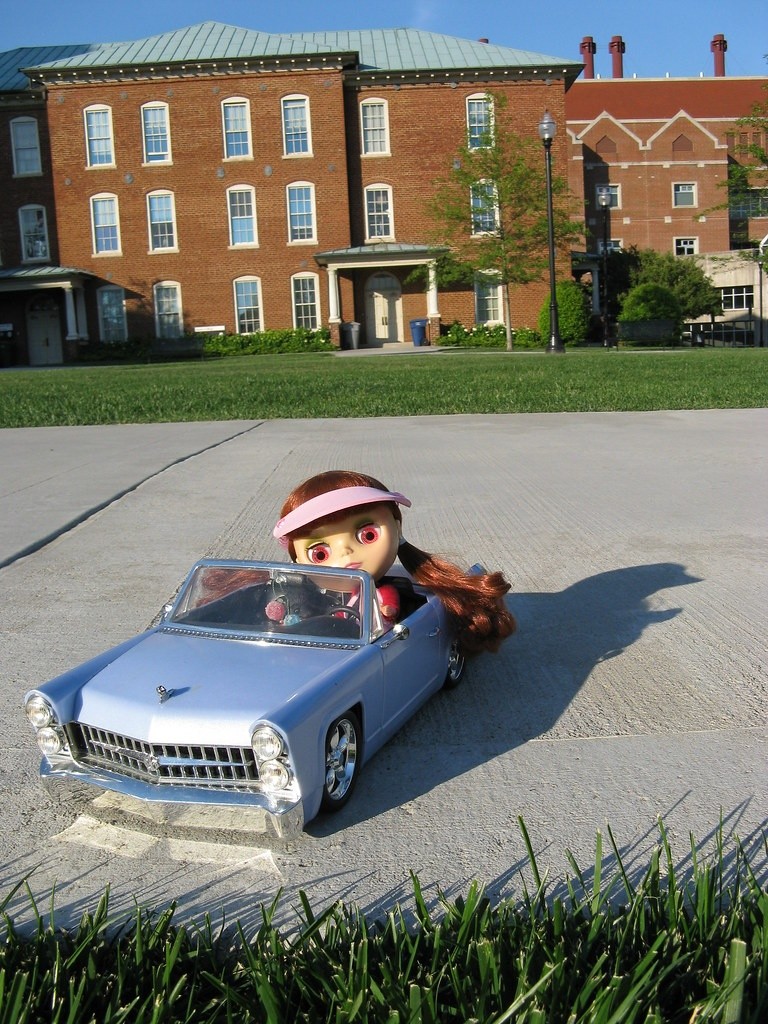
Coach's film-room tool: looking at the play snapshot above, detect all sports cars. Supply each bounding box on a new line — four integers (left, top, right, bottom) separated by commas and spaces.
22, 554, 499, 835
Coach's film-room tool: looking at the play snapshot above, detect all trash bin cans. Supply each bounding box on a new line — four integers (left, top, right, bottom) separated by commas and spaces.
339, 321, 361, 350
410, 318, 426, 346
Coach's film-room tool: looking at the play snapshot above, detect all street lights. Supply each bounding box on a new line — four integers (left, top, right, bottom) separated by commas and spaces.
598, 185, 613, 349
538, 106, 567, 355
